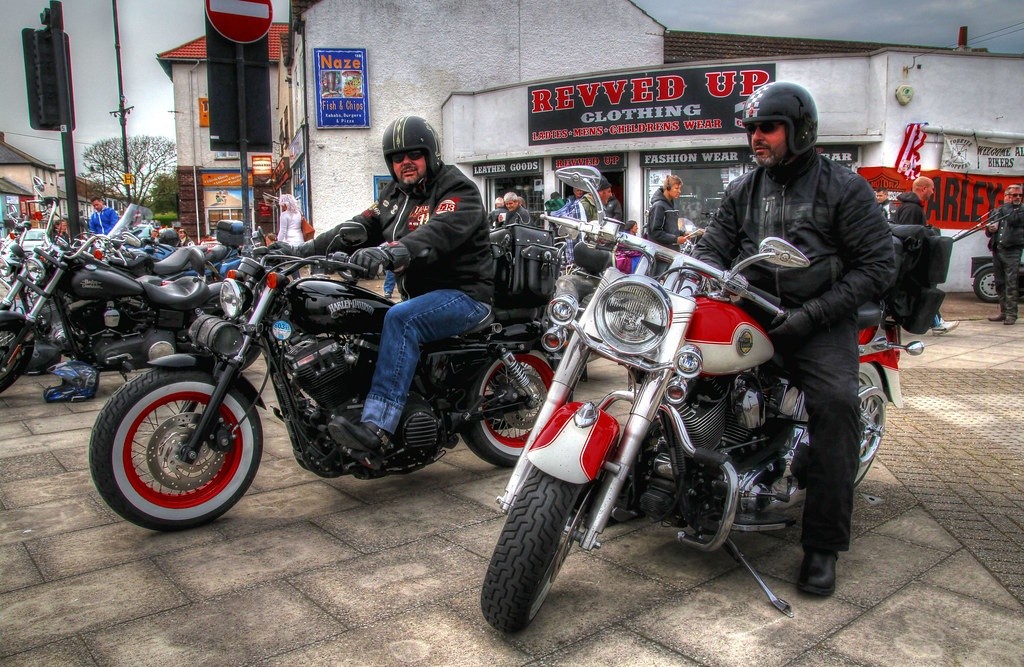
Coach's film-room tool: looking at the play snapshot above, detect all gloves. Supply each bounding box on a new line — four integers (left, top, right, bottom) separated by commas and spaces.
349, 241, 411, 281
764, 301, 829, 346
265, 237, 314, 266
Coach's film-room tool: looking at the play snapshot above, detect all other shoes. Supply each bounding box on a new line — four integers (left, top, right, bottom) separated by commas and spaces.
385, 292, 391, 298
988, 313, 1005, 322
1004, 316, 1016, 325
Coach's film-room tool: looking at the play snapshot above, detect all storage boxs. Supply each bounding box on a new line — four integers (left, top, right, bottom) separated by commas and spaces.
217, 219, 245, 246
159, 228, 182, 247
489, 222, 558, 308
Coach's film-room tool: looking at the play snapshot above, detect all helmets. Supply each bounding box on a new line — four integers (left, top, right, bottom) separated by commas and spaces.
158, 227, 179, 247
43, 360, 100, 403
742, 81, 819, 154
381, 115, 442, 182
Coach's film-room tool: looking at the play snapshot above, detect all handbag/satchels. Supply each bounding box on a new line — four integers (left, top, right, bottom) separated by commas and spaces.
301, 217, 315, 243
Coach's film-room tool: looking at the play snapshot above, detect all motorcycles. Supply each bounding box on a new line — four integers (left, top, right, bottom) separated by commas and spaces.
538, 206, 718, 380
0, 173, 266, 396
480, 163, 955, 635
87, 223, 578, 533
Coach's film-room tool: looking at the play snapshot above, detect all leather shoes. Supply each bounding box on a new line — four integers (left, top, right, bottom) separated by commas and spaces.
328, 415, 393, 470
797, 544, 840, 596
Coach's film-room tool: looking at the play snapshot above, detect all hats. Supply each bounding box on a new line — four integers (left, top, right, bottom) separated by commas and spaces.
591, 174, 612, 190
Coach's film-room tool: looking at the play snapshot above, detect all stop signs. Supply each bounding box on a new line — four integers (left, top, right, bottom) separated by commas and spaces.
205, 0, 274, 45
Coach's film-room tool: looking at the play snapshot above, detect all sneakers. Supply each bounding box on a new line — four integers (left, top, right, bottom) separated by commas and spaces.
932, 320, 960, 336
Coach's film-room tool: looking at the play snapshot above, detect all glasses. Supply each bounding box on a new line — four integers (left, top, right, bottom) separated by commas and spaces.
1005, 193, 1023, 197
391, 149, 426, 163
744, 121, 782, 136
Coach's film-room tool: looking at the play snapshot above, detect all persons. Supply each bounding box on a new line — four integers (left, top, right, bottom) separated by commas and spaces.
51, 219, 69, 244
985, 185, 1024, 327
88, 197, 196, 246
876, 176, 960, 336
265, 115, 495, 471
692, 82, 898, 596
486, 176, 691, 252
